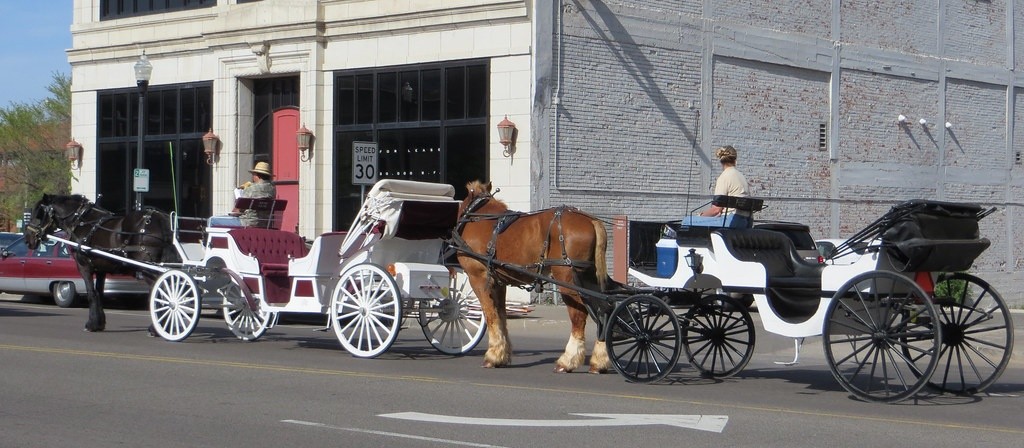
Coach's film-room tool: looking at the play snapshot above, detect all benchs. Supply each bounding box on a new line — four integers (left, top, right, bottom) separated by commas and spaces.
819, 238, 940, 306
204, 199, 289, 263
226, 228, 307, 303
710, 227, 818, 284
676, 195, 764, 273
288, 231, 368, 305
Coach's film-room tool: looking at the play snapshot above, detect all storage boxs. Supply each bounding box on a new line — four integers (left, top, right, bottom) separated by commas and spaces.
656, 238, 678, 279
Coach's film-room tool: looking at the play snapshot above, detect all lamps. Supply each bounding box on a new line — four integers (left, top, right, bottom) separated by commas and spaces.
203, 128, 218, 165
67, 137, 81, 169
496, 114, 516, 157
296, 122, 312, 162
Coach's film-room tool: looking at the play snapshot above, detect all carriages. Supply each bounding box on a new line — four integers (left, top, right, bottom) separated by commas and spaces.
23, 177, 486, 359
439, 169, 1013, 404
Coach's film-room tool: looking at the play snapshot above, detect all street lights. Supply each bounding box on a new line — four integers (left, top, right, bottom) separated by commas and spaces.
130, 50, 152, 222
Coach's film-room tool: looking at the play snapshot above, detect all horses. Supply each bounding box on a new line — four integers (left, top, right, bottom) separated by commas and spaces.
21, 193, 183, 338
436, 180, 622, 376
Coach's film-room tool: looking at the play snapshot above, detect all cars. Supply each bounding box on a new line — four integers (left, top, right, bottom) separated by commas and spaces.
0, 234, 25, 253
0, 231, 155, 308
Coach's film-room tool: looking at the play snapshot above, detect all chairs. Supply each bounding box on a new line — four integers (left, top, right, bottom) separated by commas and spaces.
35, 241, 54, 257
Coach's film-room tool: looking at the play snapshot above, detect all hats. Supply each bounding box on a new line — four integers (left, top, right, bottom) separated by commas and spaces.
715, 145, 737, 161
248, 162, 272, 176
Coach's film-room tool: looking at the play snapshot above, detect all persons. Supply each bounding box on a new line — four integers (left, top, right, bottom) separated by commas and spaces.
681, 145, 754, 229
230, 161, 276, 227
